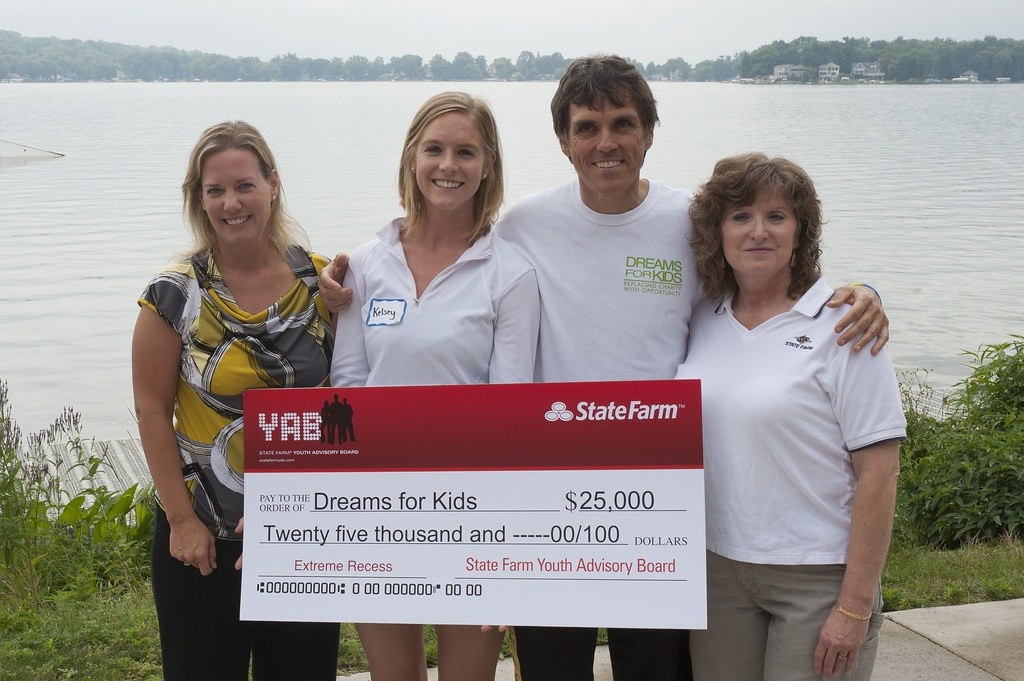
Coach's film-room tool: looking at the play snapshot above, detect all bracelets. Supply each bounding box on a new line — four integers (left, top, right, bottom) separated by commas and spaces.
849, 283, 882, 306
834, 601, 872, 622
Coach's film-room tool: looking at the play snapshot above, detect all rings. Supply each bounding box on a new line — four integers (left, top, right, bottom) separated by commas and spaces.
837, 655, 848, 661
183, 562, 192, 567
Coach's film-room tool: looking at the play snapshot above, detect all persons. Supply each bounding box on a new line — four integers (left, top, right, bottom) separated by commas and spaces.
317, 55, 891, 681
131, 119, 340, 681
673, 150, 908, 680
331, 91, 541, 681
